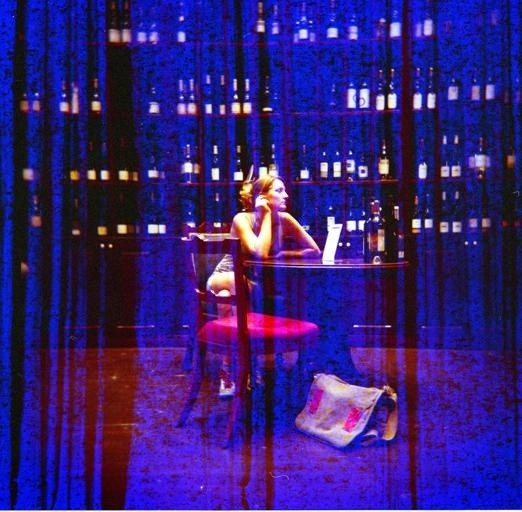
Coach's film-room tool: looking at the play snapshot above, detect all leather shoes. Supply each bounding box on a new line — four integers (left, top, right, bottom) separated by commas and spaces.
219, 370, 265, 399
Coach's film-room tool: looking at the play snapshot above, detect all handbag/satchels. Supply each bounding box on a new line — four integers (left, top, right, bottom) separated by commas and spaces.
293, 373, 385, 449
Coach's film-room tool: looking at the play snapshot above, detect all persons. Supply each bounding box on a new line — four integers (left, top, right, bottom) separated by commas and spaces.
205, 175, 322, 399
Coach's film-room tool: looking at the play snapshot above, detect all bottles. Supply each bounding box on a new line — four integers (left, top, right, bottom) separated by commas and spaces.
19, 0, 516, 264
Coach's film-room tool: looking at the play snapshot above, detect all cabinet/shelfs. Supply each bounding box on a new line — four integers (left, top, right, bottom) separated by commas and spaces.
56, 35, 516, 348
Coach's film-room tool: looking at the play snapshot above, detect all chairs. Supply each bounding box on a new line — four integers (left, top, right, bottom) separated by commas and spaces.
174, 221, 319, 448
